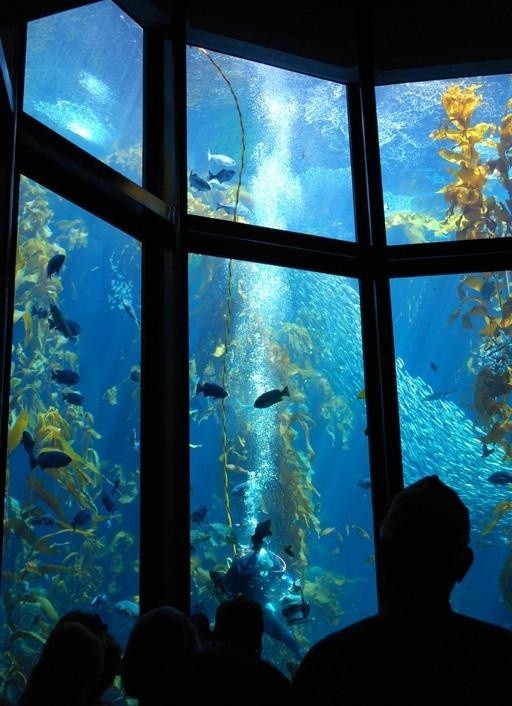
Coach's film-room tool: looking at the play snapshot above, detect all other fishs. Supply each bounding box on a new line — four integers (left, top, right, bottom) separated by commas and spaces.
188, 276, 371, 682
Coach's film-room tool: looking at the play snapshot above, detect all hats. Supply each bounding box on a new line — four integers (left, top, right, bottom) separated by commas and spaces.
376, 475, 475, 548
